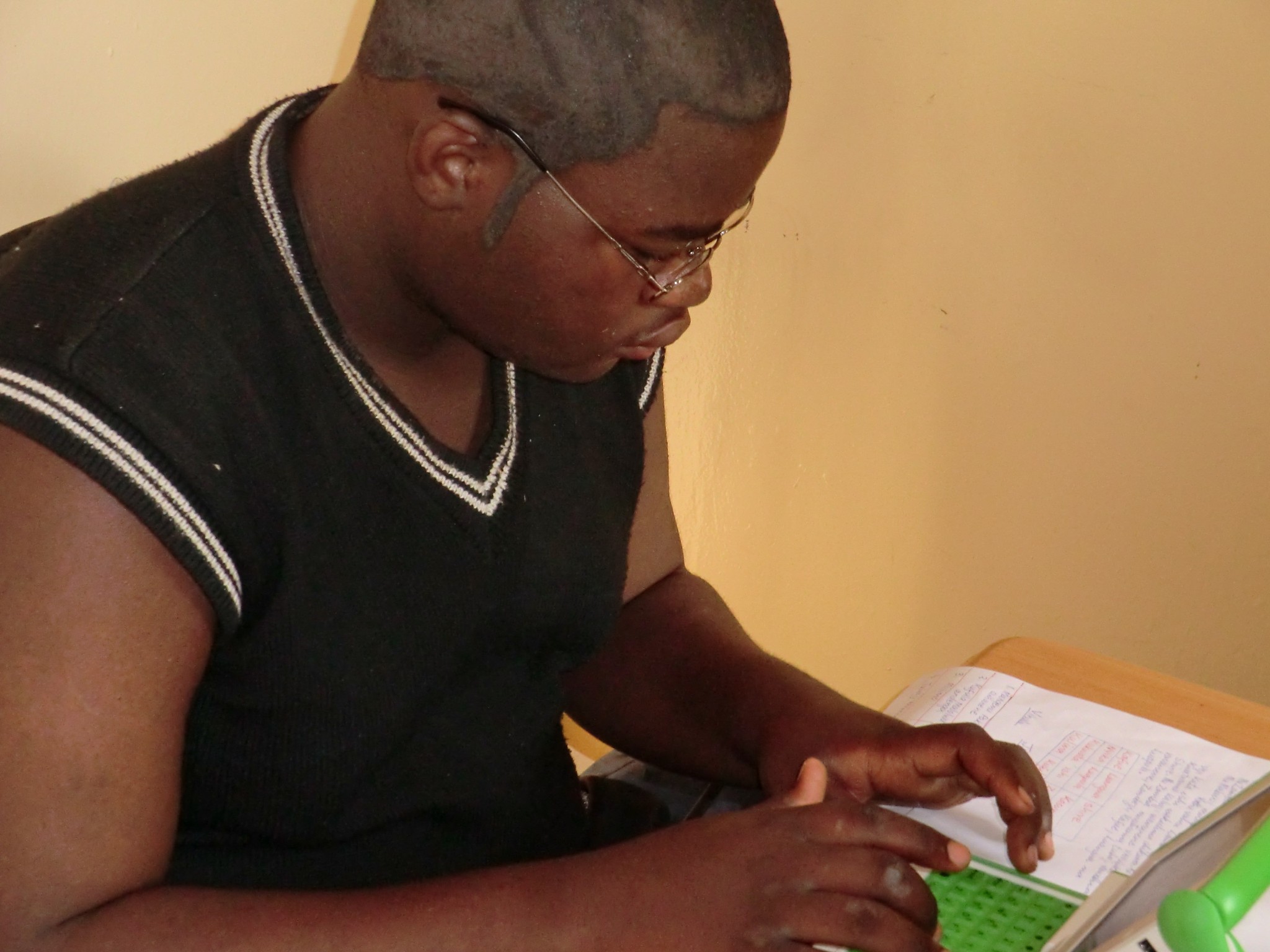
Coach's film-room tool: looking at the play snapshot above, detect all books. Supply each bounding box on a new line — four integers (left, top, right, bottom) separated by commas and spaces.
815, 667, 1270, 952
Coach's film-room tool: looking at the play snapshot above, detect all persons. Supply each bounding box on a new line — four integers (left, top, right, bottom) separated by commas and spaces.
0, 0, 1058, 952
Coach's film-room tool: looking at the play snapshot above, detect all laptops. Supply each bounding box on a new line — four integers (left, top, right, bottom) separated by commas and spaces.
809, 770, 1270, 952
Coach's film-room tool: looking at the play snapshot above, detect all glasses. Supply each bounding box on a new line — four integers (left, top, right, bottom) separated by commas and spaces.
436, 94, 756, 304
